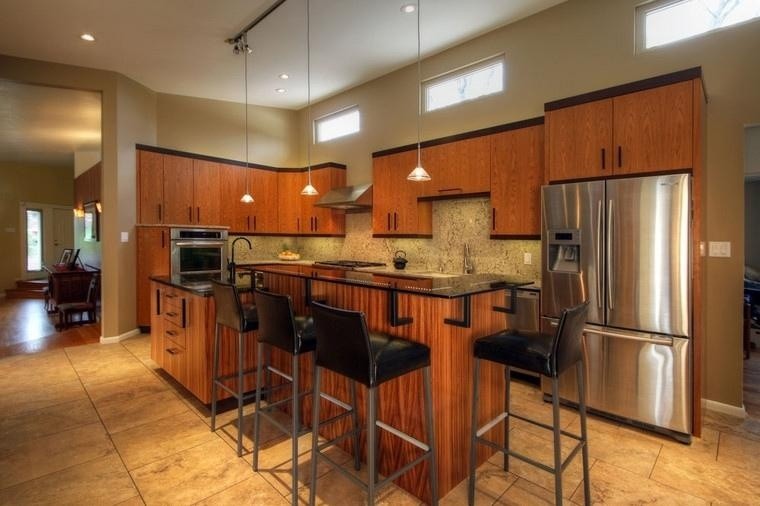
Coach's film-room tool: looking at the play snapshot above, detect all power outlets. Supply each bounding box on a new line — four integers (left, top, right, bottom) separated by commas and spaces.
710, 241, 731, 258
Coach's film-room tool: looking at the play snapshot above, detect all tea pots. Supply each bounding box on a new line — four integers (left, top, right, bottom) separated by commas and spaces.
392, 250, 408, 269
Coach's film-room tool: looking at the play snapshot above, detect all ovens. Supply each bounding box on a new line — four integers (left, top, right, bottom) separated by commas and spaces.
171, 228, 230, 282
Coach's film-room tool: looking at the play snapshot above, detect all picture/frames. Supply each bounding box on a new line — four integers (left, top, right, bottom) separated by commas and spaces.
60, 249, 72, 265
72, 249, 80, 265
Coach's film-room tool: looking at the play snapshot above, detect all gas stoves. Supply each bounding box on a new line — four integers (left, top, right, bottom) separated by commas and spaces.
315, 258, 386, 270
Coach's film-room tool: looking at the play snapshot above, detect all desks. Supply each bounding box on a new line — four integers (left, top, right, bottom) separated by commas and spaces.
43, 264, 100, 319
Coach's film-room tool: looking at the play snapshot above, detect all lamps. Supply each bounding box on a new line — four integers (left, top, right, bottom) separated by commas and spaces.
300, 0, 319, 196
240, 27, 254, 204
407, 0, 432, 182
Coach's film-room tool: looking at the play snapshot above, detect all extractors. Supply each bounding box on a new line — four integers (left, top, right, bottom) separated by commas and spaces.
314, 184, 373, 214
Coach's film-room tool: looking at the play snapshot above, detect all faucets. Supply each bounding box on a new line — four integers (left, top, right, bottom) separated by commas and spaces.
462, 242, 473, 274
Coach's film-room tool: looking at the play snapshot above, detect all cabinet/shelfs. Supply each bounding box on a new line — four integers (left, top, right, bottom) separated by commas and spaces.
545, 67, 705, 437
372, 115, 544, 240
73, 161, 101, 210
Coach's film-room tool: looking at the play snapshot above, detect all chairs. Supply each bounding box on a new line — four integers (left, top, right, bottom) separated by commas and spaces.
55, 268, 101, 326
470, 298, 592, 506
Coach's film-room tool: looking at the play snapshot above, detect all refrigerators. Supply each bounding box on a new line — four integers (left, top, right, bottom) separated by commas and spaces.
540, 173, 692, 445
